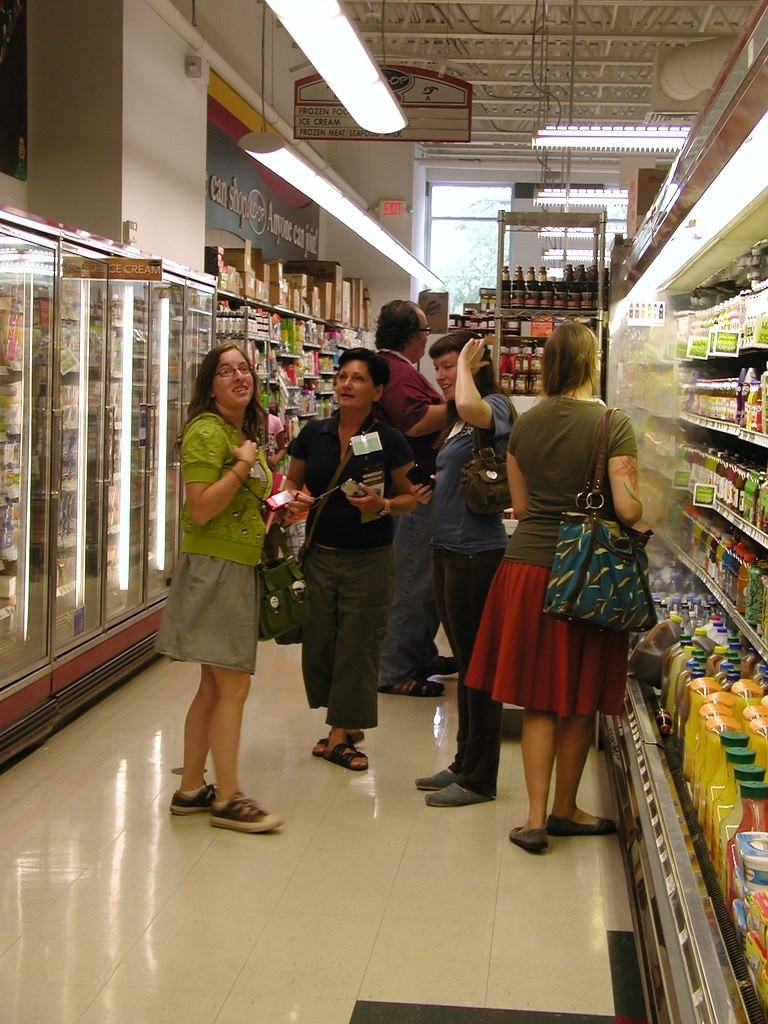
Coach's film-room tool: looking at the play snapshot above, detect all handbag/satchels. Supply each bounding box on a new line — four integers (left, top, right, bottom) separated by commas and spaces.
542, 407, 659, 634
273, 548, 307, 646
460, 393, 513, 515
253, 522, 312, 642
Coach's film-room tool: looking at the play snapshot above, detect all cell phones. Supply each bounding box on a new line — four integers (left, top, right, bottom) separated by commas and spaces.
341, 478, 367, 498
264, 490, 296, 512
404, 464, 436, 490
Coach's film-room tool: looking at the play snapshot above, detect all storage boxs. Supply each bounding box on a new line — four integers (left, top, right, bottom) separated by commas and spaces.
205, 239, 371, 548
418, 289, 450, 334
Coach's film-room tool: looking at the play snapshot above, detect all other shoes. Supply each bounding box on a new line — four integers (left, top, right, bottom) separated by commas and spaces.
508, 826, 549, 850
415, 769, 458, 790
544, 813, 617, 836
424, 782, 493, 807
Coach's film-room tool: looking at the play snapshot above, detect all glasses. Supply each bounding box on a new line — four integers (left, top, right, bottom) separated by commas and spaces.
214, 364, 255, 378
418, 327, 431, 336
596, 349, 604, 359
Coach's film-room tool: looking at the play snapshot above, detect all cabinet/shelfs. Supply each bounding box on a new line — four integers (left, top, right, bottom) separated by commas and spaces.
218, 290, 372, 478
495, 208, 607, 418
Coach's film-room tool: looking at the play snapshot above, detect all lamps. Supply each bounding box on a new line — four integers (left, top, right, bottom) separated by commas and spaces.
531, 0, 694, 277
239, 1, 444, 288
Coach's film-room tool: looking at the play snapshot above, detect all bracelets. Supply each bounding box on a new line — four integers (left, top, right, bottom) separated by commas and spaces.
238, 460, 252, 468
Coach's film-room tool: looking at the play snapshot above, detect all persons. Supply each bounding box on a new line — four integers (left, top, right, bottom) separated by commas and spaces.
464, 323, 642, 850
416, 331, 517, 807
154, 343, 304, 834
267, 413, 287, 469
372, 297, 460, 698
279, 348, 417, 769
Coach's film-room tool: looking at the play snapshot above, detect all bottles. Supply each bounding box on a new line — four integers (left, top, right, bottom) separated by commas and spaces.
629, 303, 663, 319
503, 266, 548, 286
689, 279, 768, 351
736, 366, 761, 433
628, 592, 767, 821
562, 265, 608, 310
501, 346, 544, 379
703, 732, 768, 922
691, 378, 739, 423
679, 441, 768, 533
680, 504, 768, 645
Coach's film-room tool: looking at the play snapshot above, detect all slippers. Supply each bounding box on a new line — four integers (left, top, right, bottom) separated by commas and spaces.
312, 730, 365, 757
323, 743, 368, 771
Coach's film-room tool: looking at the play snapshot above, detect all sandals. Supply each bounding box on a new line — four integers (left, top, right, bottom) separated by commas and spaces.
420, 655, 459, 679
378, 677, 445, 697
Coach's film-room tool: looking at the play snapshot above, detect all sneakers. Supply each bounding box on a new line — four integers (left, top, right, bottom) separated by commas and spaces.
169, 780, 220, 815
207, 792, 283, 834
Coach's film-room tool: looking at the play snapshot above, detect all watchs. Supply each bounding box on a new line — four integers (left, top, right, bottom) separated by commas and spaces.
379, 500, 391, 515
